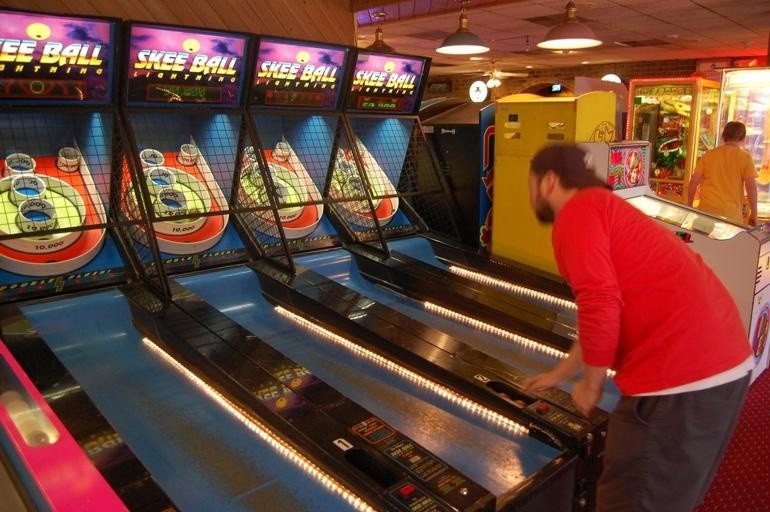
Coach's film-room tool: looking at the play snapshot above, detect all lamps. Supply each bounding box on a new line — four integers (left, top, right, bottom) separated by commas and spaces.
366, 13, 396, 53
536, 0, 602, 50
486, 71, 502, 88
436, 0, 490, 55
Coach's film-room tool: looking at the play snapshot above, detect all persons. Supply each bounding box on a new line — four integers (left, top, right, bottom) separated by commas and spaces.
689, 118, 760, 230
518, 142, 757, 510
